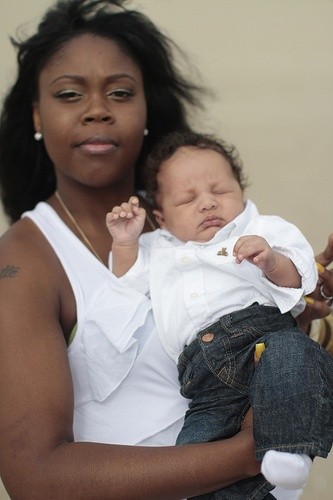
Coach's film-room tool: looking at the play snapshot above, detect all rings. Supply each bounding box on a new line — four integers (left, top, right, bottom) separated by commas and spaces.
319, 284, 333, 301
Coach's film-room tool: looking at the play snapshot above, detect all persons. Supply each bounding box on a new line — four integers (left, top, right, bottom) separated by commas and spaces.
105, 128, 333, 499
0, 0, 333, 500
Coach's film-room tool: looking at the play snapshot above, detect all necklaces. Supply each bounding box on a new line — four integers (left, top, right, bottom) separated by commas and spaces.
53, 189, 159, 265
